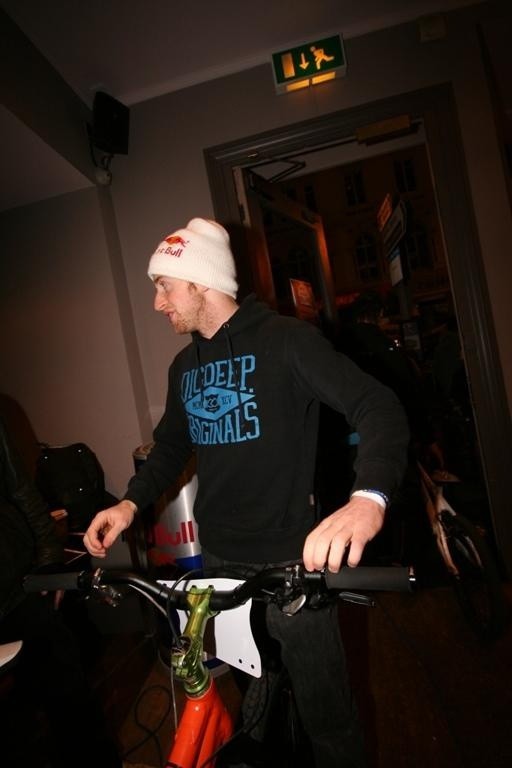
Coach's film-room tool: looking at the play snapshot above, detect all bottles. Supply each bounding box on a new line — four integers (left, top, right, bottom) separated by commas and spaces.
147, 217, 239, 300
0, 640, 23, 667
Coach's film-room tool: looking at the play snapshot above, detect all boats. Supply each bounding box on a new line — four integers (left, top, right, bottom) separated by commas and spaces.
89, 88, 131, 154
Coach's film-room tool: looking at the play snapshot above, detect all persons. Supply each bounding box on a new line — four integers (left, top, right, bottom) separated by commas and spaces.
82, 214, 413, 767
